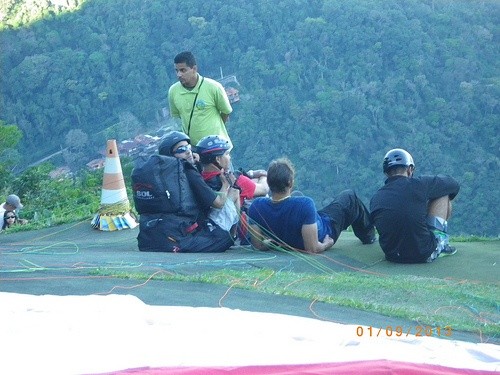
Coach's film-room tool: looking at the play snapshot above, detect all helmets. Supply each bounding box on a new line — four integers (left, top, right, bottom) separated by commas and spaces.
195, 135, 234, 166
383, 148, 415, 173
158, 131, 191, 156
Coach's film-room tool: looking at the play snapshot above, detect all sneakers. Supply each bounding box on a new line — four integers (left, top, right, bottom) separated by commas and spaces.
438, 244, 457, 258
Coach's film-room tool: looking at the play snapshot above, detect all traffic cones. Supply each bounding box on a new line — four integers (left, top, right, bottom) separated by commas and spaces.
91, 140, 133, 231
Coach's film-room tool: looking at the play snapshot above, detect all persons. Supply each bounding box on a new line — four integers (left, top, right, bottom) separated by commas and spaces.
130, 132, 239, 253
247, 159, 376, 254
369, 148, 460, 264
0, 193, 28, 231
196, 135, 271, 246
168, 51, 234, 152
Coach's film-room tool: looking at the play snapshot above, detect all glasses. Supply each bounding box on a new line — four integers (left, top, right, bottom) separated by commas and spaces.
172, 144, 192, 154
4, 215, 15, 219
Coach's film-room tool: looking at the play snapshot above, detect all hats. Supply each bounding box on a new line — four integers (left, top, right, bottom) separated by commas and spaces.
6, 195, 23, 208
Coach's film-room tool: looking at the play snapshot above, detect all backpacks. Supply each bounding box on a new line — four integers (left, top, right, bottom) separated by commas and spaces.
130, 153, 201, 252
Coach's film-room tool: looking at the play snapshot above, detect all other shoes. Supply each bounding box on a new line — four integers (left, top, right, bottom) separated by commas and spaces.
241, 237, 251, 245
363, 237, 376, 244
